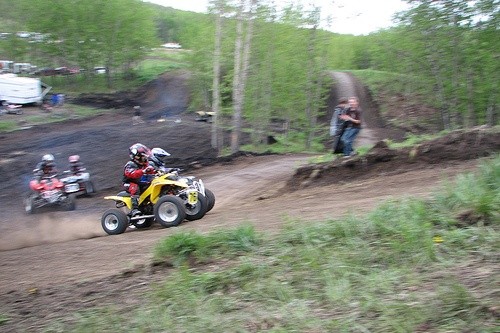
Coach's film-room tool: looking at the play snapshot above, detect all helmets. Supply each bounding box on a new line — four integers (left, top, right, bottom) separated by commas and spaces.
42, 153, 56, 161
149, 148, 171, 166
129, 143, 153, 165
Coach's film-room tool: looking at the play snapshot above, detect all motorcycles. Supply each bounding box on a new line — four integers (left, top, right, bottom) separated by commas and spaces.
58, 167, 94, 199
23, 172, 75, 214
101, 170, 216, 235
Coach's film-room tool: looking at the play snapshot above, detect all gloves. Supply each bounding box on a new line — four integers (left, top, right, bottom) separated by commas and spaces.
143, 166, 155, 174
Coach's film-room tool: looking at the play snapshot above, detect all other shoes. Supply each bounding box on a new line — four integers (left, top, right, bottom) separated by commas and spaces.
131, 209, 142, 217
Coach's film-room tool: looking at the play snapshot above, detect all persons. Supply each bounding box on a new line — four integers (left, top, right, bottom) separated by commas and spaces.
33, 154, 58, 182
147, 147, 181, 174
68, 155, 85, 176
121, 143, 162, 217
330, 96, 363, 156
